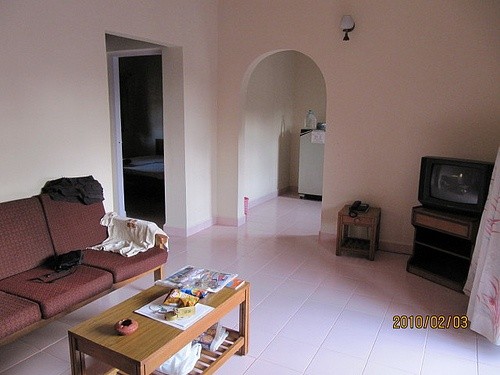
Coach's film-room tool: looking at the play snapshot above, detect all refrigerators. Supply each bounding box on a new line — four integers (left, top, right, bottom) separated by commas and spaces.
297, 128, 325, 200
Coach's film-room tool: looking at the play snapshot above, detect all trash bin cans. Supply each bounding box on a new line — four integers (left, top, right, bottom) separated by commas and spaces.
244, 196, 249, 216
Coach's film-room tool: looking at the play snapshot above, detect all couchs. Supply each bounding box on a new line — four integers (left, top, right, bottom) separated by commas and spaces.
0, 179, 167, 348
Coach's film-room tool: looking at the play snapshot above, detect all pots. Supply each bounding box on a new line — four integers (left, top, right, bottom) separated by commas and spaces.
317, 121, 327, 130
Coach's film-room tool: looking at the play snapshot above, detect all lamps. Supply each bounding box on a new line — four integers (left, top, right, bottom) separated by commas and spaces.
341, 15, 355, 40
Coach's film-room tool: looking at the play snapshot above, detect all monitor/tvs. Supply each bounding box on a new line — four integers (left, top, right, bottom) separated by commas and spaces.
417, 156, 496, 218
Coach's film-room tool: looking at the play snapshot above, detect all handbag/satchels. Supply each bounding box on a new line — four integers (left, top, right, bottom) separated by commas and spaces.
30, 250, 81, 283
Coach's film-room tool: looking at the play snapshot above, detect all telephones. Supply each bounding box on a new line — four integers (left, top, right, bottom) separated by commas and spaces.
349, 200, 369, 218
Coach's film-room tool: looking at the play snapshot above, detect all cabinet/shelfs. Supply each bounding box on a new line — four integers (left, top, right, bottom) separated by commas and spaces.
406, 205, 482, 295
335, 204, 381, 261
